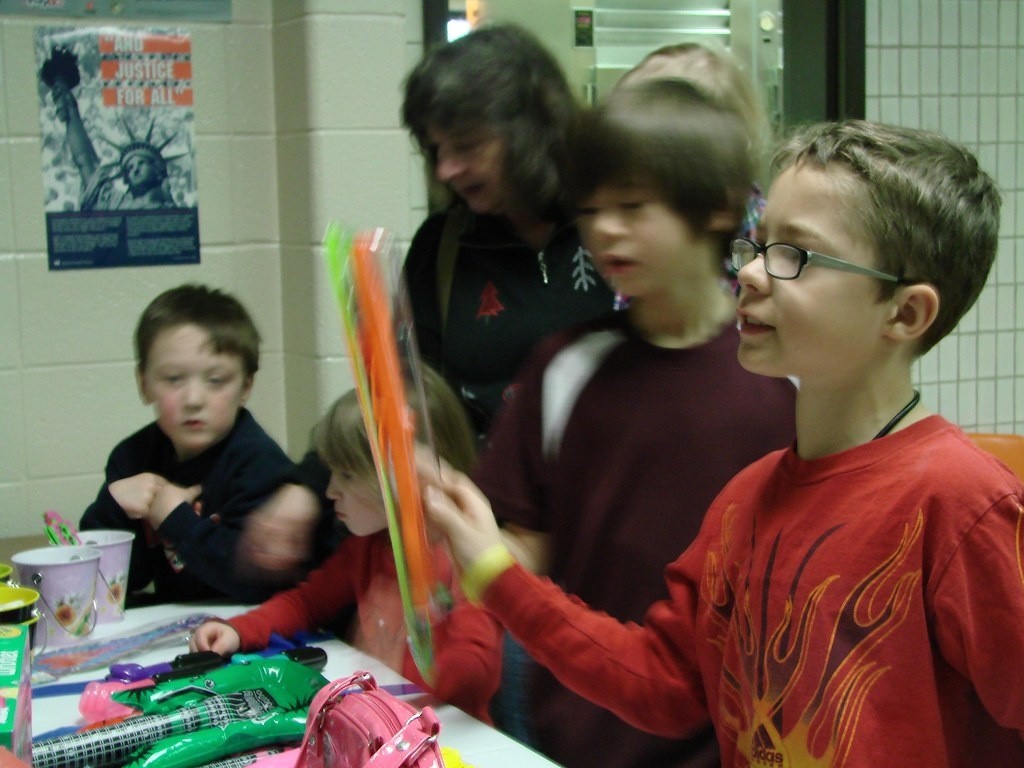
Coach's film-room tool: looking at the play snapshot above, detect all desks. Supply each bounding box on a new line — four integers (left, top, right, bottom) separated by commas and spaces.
33, 601, 560, 766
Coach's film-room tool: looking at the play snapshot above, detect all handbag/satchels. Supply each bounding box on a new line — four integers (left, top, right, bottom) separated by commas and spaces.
291, 668, 445, 768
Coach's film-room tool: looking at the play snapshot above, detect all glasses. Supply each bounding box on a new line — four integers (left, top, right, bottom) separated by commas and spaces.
728, 233, 899, 284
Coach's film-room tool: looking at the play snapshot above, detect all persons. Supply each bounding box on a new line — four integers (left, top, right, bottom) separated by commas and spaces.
412, 117, 1024, 768
484, 41, 798, 768
78, 284, 299, 606
390, 19, 631, 768
189, 357, 502, 727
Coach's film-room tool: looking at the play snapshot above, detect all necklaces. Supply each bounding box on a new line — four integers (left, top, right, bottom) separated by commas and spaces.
868, 388, 921, 442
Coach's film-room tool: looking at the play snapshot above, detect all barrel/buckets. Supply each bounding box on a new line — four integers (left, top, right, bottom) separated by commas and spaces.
12, 546, 103, 646
49, 530, 135, 623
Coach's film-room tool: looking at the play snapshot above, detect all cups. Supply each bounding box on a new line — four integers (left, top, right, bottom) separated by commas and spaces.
0, 563, 40, 652
